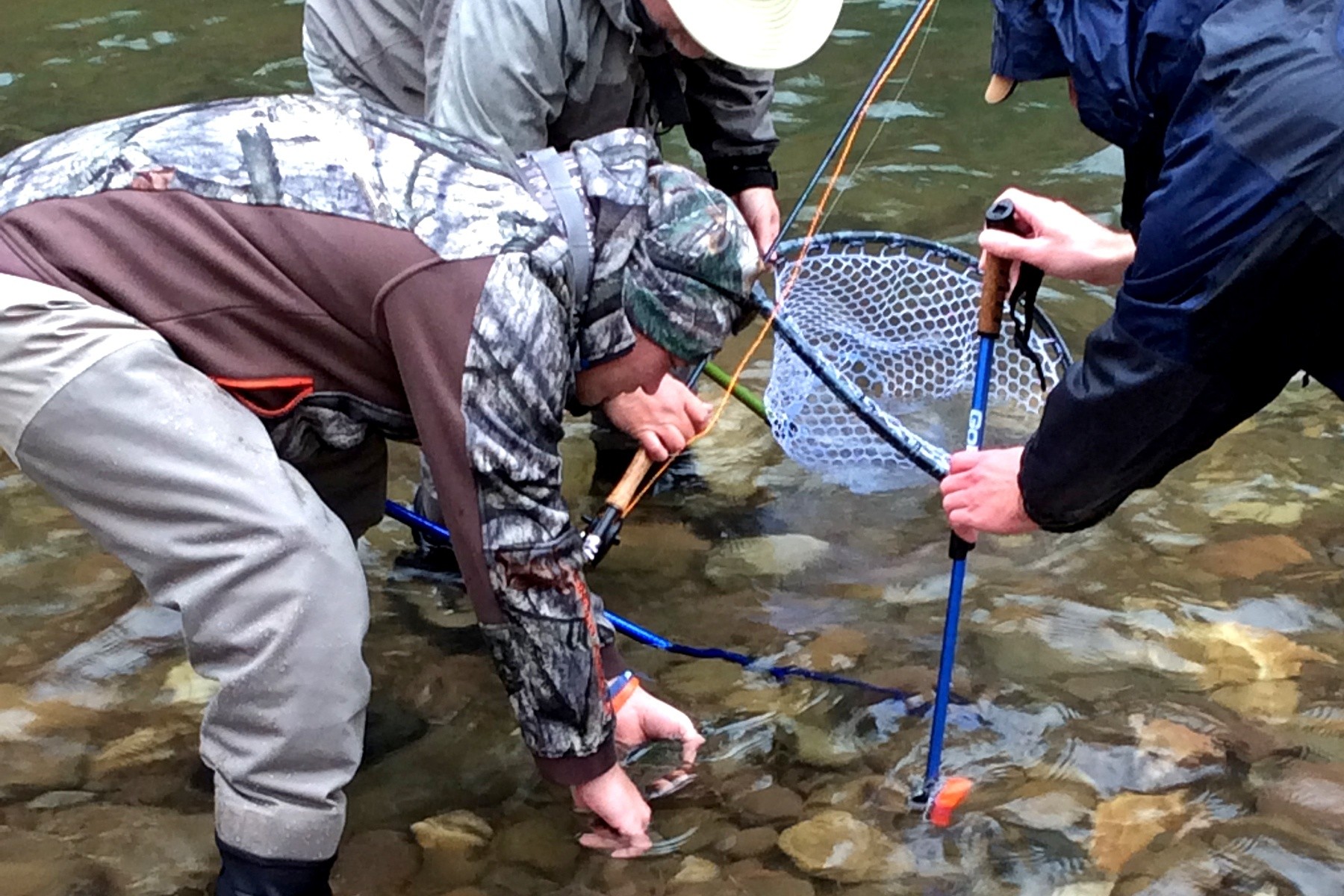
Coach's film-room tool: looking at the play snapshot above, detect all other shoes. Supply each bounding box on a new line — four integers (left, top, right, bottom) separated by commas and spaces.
409, 485, 466, 587
589, 439, 709, 507
354, 703, 429, 768
211, 834, 339, 895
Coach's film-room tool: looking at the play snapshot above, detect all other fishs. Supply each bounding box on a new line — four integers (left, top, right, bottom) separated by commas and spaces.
573, 714, 794, 858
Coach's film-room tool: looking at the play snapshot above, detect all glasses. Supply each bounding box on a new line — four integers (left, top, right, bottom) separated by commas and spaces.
649, 257, 761, 336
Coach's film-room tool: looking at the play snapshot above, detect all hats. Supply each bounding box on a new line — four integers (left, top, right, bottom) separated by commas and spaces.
623, 163, 763, 365
667, 1, 843, 70
981, 73, 1018, 104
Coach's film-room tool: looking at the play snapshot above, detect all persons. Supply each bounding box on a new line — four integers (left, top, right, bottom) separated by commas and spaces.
0, 94, 765, 896
941, 0, 1344, 541
303, 0, 845, 549
